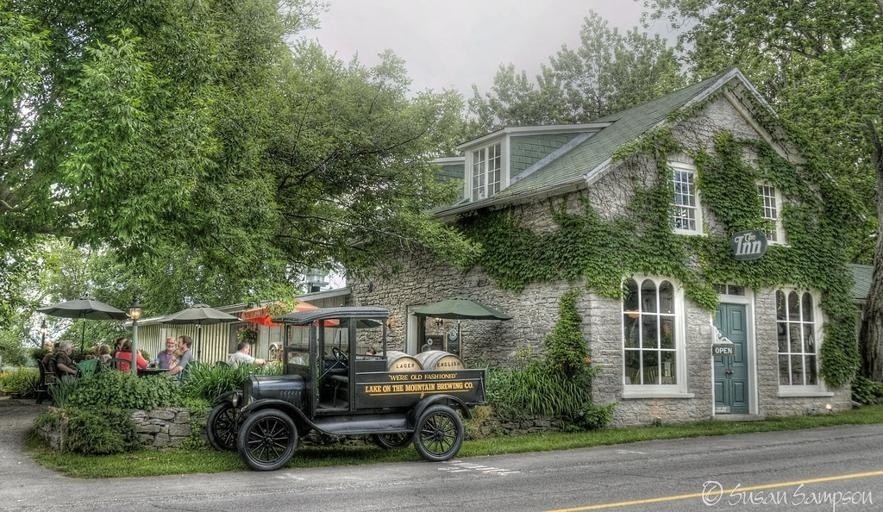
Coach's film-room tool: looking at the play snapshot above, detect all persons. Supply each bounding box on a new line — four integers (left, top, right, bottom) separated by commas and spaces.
268, 342, 282, 364
112, 337, 127, 369
287, 345, 305, 365
169, 335, 196, 375
41, 342, 59, 368
97, 346, 110, 363
228, 339, 267, 367
154, 337, 180, 370
116, 340, 149, 372
49, 340, 76, 381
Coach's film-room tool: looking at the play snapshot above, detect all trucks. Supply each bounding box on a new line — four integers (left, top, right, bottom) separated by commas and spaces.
205, 305, 491, 474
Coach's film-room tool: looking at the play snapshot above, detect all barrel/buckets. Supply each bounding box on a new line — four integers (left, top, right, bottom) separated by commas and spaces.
371, 350, 424, 371
413, 349, 467, 370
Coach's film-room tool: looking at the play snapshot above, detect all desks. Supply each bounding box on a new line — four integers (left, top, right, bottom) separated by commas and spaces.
138, 368, 170, 371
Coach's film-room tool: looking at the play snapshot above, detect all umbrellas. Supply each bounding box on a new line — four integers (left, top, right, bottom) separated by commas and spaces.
36, 292, 129, 353
411, 293, 513, 359
158, 302, 240, 368
241, 298, 340, 342
330, 317, 384, 331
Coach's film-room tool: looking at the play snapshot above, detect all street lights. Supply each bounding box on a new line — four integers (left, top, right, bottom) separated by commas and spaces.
126, 297, 144, 382
37, 318, 47, 349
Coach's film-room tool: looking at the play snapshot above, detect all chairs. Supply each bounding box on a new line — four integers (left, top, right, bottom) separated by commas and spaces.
331, 356, 382, 407
105, 358, 132, 373
36, 359, 56, 406
